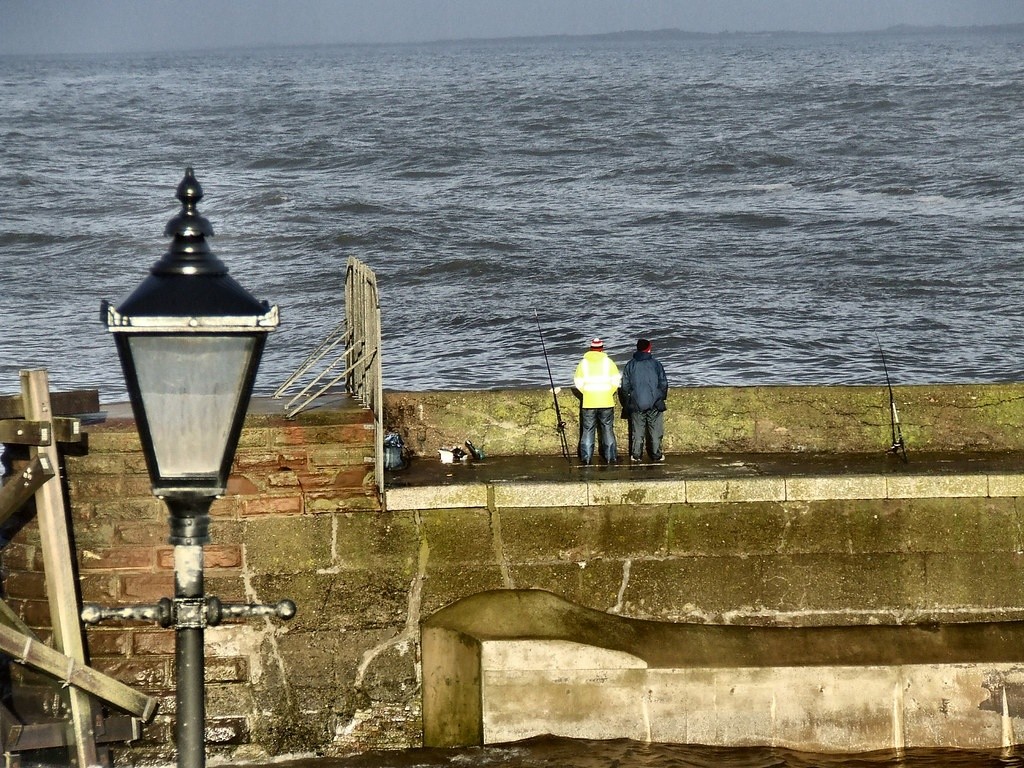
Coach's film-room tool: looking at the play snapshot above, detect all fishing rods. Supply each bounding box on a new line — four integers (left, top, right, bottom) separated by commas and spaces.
533, 304, 572, 462
876, 331, 906, 454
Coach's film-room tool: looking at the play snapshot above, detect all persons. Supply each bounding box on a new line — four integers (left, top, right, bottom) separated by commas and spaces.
574, 336, 622, 465
618, 339, 668, 462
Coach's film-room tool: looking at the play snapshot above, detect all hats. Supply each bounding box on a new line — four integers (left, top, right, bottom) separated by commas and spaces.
591, 337, 604, 351
636, 339, 651, 352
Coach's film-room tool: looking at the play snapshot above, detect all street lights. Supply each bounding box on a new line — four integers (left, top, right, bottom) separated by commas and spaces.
79, 167, 298, 768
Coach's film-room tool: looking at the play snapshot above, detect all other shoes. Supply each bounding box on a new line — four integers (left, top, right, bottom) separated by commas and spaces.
630, 455, 643, 462
583, 460, 590, 464
654, 454, 666, 462
607, 457, 617, 463
